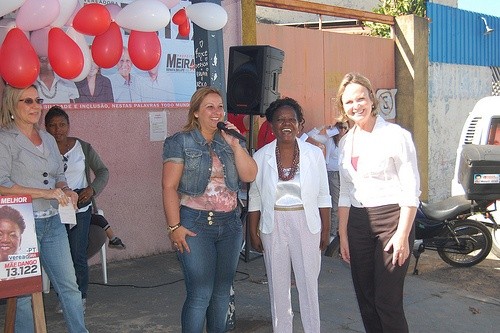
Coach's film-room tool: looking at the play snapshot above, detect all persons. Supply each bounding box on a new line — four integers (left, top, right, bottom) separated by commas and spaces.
247, 98, 332, 333
45, 106, 109, 315
258, 122, 275, 147
336, 71, 422, 333
307, 119, 349, 237
0, 85, 89, 333
0, 205, 26, 261
91, 215, 126, 250
161, 88, 253, 333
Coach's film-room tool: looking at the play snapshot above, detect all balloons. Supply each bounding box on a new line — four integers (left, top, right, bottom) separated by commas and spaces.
50, 0, 78, 28
91, 0, 228, 69
48, 28, 84, 79
0, 28, 40, 89
0, 0, 26, 17
16, 0, 60, 31
73, 4, 110, 37
54, 26, 92, 83
31, 25, 53, 61
65, 0, 81, 26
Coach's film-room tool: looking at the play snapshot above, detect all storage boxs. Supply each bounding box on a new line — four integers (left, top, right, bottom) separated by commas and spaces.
458, 144, 500, 199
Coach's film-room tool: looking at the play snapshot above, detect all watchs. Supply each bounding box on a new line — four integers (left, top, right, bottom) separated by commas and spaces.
167, 223, 181, 233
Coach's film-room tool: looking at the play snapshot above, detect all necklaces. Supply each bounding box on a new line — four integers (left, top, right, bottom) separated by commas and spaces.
274, 141, 298, 181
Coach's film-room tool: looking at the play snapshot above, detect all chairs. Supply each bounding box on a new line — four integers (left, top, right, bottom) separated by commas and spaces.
43, 196, 107, 293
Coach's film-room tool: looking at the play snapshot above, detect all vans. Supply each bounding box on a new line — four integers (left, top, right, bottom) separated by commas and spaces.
452, 96, 500, 259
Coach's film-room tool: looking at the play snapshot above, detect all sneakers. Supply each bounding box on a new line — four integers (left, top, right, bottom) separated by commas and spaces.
108, 237, 126, 249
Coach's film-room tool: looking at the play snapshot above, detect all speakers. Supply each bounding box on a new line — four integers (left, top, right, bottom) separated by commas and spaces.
227, 45, 285, 115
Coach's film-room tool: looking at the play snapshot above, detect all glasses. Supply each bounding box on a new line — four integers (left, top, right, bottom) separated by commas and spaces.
339, 126, 347, 130
18, 98, 44, 104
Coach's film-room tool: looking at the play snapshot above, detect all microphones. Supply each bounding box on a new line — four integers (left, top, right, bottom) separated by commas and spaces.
216, 122, 247, 141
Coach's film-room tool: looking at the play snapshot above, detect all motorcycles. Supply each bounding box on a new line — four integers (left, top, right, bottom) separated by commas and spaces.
325, 195, 496, 276
